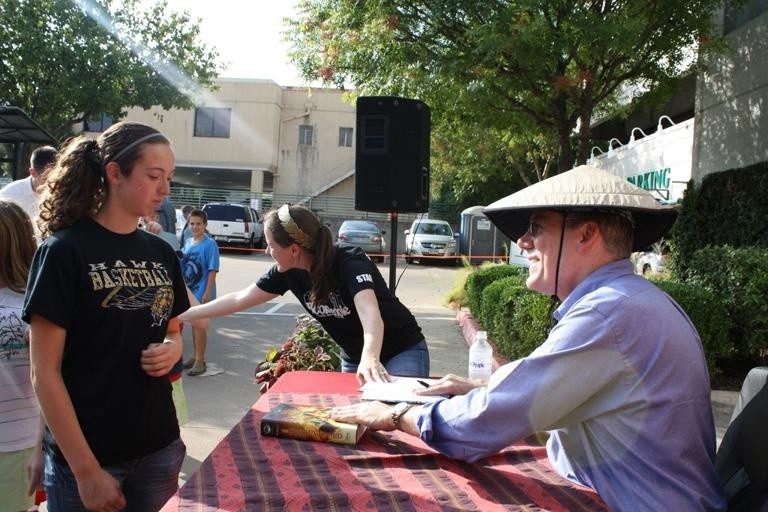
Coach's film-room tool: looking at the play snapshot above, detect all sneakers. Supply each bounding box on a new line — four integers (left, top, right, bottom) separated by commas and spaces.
183, 358, 195, 368
187, 362, 207, 375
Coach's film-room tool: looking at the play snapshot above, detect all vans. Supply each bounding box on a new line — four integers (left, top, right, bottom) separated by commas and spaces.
199, 203, 263, 253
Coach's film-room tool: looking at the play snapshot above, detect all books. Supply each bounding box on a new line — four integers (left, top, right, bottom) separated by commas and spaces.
261, 402, 368, 444
360, 379, 453, 404
357, 374, 440, 392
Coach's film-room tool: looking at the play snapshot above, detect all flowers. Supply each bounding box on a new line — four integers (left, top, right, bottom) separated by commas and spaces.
252, 313, 339, 394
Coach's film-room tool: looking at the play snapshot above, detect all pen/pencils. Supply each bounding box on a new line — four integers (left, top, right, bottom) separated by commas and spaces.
417, 380, 452, 399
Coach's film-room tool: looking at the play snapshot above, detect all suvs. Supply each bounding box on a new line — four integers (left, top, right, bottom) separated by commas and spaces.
403, 219, 459, 266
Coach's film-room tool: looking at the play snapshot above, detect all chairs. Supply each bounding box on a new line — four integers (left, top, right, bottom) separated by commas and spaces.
713, 364, 768, 511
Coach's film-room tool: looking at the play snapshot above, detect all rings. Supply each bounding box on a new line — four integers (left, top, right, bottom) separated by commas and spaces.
378, 370, 385, 376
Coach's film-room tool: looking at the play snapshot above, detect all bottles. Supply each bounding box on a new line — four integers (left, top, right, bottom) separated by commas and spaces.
468, 330, 493, 380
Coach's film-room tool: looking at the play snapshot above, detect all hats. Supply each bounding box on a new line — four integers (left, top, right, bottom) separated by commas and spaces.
482, 164, 678, 252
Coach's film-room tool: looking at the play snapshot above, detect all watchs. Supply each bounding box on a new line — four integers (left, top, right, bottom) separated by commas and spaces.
391, 401, 412, 432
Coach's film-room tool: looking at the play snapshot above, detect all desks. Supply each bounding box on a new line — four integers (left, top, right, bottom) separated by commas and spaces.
159, 371, 611, 512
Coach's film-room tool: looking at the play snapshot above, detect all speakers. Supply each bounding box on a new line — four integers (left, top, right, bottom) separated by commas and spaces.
355, 96, 430, 213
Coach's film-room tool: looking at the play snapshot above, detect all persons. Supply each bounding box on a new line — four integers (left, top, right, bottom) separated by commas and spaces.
1, 144, 59, 245
322, 163, 721, 511
0, 200, 45, 512
135, 196, 220, 376
24, 120, 190, 512
177, 203, 430, 387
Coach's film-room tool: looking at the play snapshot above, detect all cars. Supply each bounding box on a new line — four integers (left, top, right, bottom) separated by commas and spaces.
336, 220, 386, 261
629, 249, 670, 277
